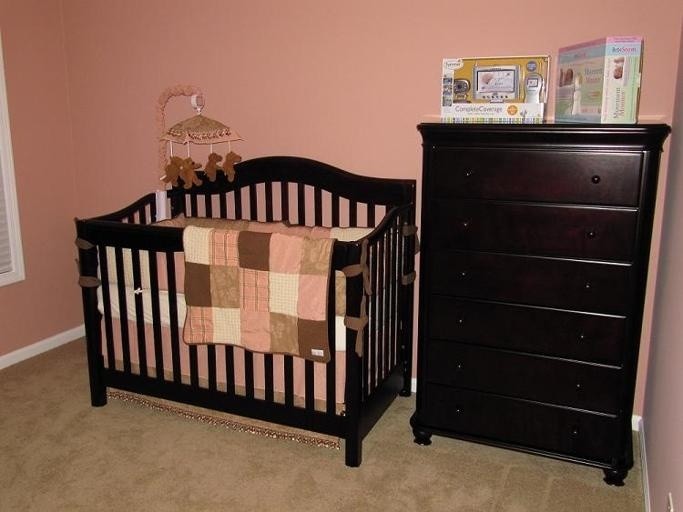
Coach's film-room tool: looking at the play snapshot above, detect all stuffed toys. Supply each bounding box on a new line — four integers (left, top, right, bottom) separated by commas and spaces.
161, 92, 242, 189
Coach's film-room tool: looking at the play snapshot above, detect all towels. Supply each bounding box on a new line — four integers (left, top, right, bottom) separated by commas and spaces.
182, 225, 339, 363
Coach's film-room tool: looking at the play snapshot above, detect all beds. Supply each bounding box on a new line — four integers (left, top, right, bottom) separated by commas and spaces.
73, 153, 421, 467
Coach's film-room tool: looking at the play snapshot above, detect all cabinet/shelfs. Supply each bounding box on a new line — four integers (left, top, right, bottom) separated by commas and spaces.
409, 120, 672, 486
